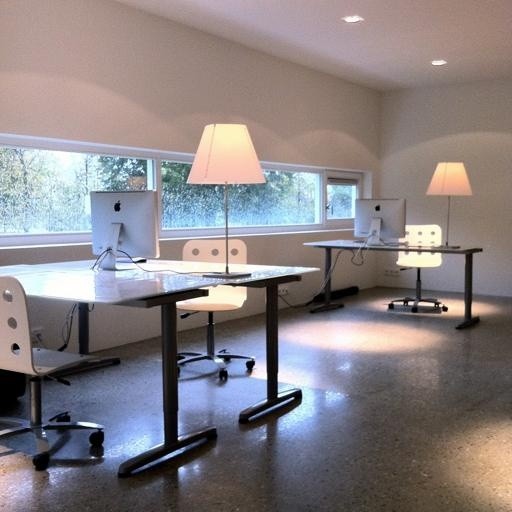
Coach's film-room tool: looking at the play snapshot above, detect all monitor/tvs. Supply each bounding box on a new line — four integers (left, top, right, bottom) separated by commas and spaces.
90, 190, 160, 271
354, 198, 406, 246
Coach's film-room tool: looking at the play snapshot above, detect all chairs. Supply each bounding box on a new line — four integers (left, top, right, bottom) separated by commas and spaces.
174, 237, 254, 381
2, 276, 121, 472
388, 224, 447, 314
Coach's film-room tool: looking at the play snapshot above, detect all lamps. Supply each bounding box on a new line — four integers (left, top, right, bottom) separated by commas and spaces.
424, 161, 474, 249
185, 123, 268, 279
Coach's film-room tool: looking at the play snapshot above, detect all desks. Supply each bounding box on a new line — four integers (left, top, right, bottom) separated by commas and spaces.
2, 257, 320, 452
302, 236, 484, 330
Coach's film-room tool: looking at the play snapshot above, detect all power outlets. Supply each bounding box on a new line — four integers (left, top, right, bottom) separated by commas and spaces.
277, 288, 290, 296
32, 330, 47, 342
